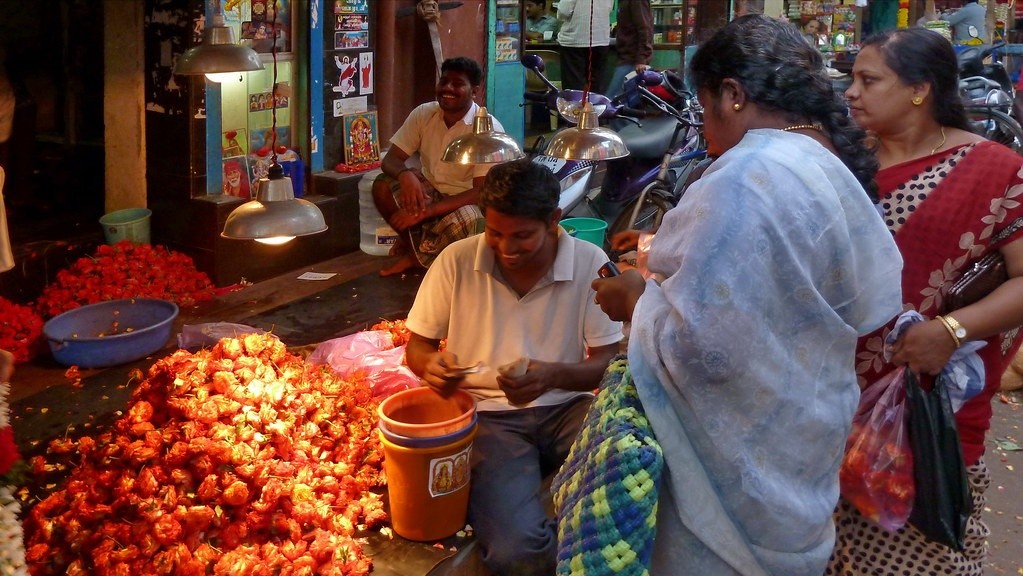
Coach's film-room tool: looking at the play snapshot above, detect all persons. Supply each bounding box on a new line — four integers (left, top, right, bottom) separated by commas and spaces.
940, 0, 988, 45
795, 17, 822, 49
224, 160, 249, 202
406, 161, 630, 576
273, 93, 288, 108
266, 94, 273, 109
524, 0, 555, 44
556, 0, 620, 134
370, 57, 506, 276
605, 0, 654, 109
821, 27, 1023, 576
257, 95, 266, 110
593, 13, 886, 576
250, 95, 258, 111
223, 138, 244, 157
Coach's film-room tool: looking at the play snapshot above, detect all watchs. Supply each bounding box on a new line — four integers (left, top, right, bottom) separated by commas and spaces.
945, 315, 970, 346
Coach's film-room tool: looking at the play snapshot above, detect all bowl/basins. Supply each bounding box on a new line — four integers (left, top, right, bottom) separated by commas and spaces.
42, 297, 178, 369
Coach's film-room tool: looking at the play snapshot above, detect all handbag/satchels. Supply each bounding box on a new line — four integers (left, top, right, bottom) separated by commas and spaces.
549, 352, 665, 576
906, 369, 975, 553
837, 365, 914, 534
947, 251, 1009, 317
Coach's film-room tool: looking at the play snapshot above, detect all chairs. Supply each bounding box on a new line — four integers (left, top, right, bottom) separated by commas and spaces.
524, 50, 562, 131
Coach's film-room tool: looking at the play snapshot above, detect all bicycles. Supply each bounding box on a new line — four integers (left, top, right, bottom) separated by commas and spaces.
963, 103, 1023, 156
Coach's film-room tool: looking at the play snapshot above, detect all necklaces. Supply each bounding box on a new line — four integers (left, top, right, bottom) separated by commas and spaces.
929, 122, 951, 157
772, 121, 829, 136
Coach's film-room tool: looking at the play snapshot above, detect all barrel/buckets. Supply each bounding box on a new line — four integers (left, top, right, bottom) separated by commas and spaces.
377, 383, 478, 542
98, 207, 152, 246
559, 218, 608, 250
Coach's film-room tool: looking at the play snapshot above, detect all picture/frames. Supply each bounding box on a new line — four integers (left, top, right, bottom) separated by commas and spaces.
221, 154, 252, 203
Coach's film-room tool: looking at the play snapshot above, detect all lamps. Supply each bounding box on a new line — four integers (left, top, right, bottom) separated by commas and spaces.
543, 0, 631, 160
220, 0, 328, 245
441, 0, 525, 165
173, 0, 266, 82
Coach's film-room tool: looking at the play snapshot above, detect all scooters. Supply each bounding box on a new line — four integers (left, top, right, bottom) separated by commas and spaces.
520, 53, 715, 247
952, 25, 1016, 146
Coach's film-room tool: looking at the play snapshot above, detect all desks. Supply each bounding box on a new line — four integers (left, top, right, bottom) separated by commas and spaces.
527, 41, 559, 45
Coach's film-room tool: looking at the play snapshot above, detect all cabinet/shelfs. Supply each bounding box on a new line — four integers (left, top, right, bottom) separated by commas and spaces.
649, 0, 701, 49
487, 0, 527, 65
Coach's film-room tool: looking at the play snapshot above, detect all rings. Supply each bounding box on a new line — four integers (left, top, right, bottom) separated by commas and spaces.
594, 298, 600, 304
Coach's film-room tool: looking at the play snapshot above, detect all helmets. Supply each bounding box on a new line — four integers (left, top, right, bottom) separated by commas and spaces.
643, 71, 688, 104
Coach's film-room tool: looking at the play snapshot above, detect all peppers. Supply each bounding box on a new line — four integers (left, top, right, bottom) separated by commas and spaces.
0, 241, 215, 362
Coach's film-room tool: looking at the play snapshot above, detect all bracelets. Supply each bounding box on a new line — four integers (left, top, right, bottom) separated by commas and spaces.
936, 316, 961, 349
